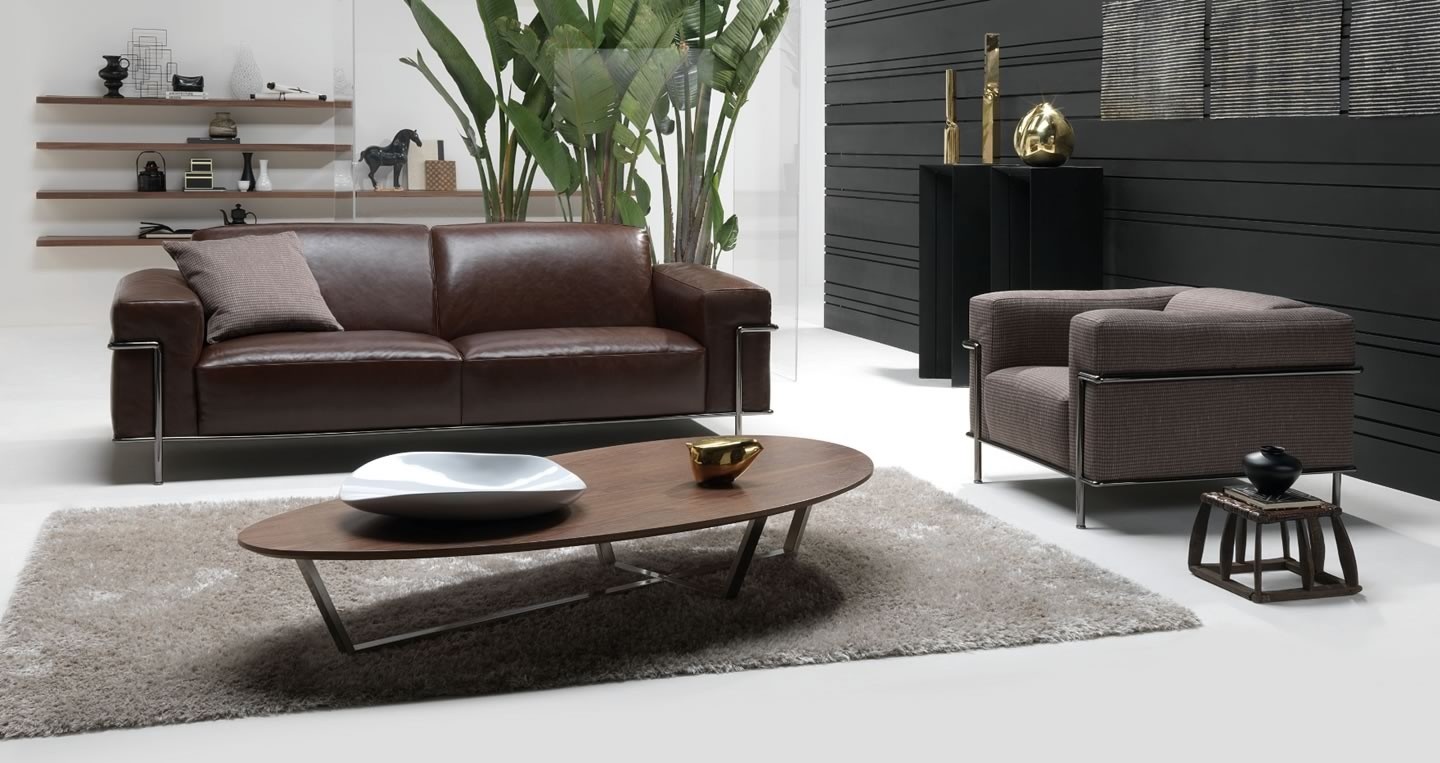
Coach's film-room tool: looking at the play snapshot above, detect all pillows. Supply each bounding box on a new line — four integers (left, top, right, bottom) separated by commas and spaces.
156, 231, 345, 347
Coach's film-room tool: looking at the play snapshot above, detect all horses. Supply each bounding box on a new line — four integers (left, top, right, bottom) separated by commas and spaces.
358, 129, 423, 191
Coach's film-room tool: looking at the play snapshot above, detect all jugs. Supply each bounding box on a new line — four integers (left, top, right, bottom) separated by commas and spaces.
136, 151, 166, 191
220, 204, 257, 225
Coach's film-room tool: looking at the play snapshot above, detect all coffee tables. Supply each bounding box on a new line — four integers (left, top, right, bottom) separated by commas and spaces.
240, 437, 874, 658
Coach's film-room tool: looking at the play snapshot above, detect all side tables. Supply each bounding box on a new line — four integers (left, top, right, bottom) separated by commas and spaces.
1189, 493, 1365, 601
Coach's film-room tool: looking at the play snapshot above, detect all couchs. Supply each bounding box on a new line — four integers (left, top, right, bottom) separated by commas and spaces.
965, 285, 1365, 528
109, 222, 779, 486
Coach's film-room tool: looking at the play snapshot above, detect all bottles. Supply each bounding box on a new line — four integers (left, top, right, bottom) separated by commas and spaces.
210, 112, 237, 140
255, 159, 273, 191
241, 152, 256, 192
1242, 445, 1303, 496
99, 56, 130, 98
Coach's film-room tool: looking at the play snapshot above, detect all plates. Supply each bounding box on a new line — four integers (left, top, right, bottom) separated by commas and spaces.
339, 451, 587, 522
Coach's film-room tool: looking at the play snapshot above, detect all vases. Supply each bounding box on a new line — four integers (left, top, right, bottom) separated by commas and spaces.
256, 159, 273, 191
241, 151, 256, 192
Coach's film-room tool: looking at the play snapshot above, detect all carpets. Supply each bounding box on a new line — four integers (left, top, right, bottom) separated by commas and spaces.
0, 468, 1202, 741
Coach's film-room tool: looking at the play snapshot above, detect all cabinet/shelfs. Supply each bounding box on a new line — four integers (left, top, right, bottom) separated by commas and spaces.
35, 96, 643, 248
918, 164, 1103, 387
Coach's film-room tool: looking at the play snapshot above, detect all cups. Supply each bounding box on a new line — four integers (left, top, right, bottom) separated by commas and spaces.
236, 181, 250, 193
